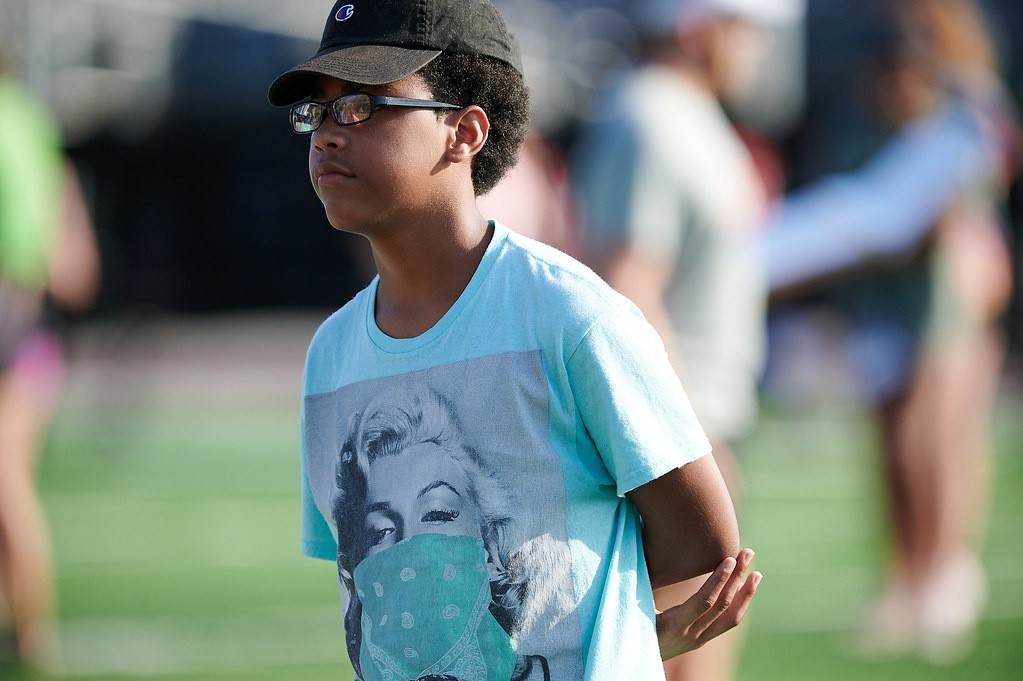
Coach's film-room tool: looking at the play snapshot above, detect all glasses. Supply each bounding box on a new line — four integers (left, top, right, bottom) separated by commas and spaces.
288, 88, 464, 134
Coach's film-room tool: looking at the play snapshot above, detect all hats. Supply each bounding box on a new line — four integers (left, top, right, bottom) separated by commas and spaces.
268, 0, 523, 123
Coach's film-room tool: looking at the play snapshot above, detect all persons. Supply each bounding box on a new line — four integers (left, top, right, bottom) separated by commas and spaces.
0, 75, 95, 681
269, 0, 763, 681
479, 1, 1009, 681
